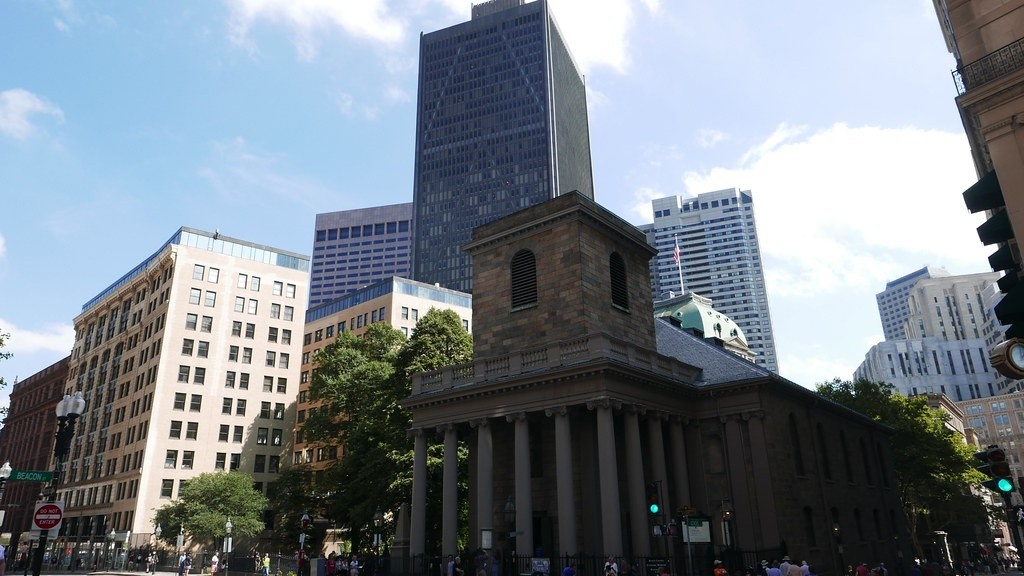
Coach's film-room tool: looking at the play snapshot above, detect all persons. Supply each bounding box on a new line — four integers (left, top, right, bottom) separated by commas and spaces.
211, 551, 226, 576
319, 551, 389, 576
911, 555, 1015, 576
847, 562, 888, 576
178, 552, 192, 576
658, 567, 669, 576
447, 554, 464, 576
256, 552, 270, 576
604, 556, 618, 576
128, 551, 159, 575
561, 563, 577, 576
75, 555, 81, 570
472, 547, 489, 576
51, 556, 57, 570
0, 545, 5, 576
714, 556, 818, 576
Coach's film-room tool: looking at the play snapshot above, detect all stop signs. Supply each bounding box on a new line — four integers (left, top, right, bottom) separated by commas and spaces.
31, 500, 64, 531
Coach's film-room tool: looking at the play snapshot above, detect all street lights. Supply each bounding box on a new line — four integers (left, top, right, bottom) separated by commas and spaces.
830, 522, 849, 576
30, 388, 87, 575
935, 530, 957, 571
224, 517, 233, 576
0, 461, 13, 505
502, 494, 520, 576
721, 498, 735, 551
151, 522, 162, 575
297, 508, 309, 576
106, 528, 116, 572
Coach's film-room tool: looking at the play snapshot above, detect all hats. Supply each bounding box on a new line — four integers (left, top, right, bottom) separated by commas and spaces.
714, 560, 722, 565
782, 556, 790, 561
772, 561, 780, 567
761, 560, 768, 565
801, 561, 807, 565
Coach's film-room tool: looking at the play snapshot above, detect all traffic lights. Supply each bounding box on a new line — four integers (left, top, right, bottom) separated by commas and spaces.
960, 168, 1024, 340
653, 524, 662, 537
974, 450, 997, 495
647, 482, 668, 518
987, 447, 1018, 497
668, 523, 679, 537
